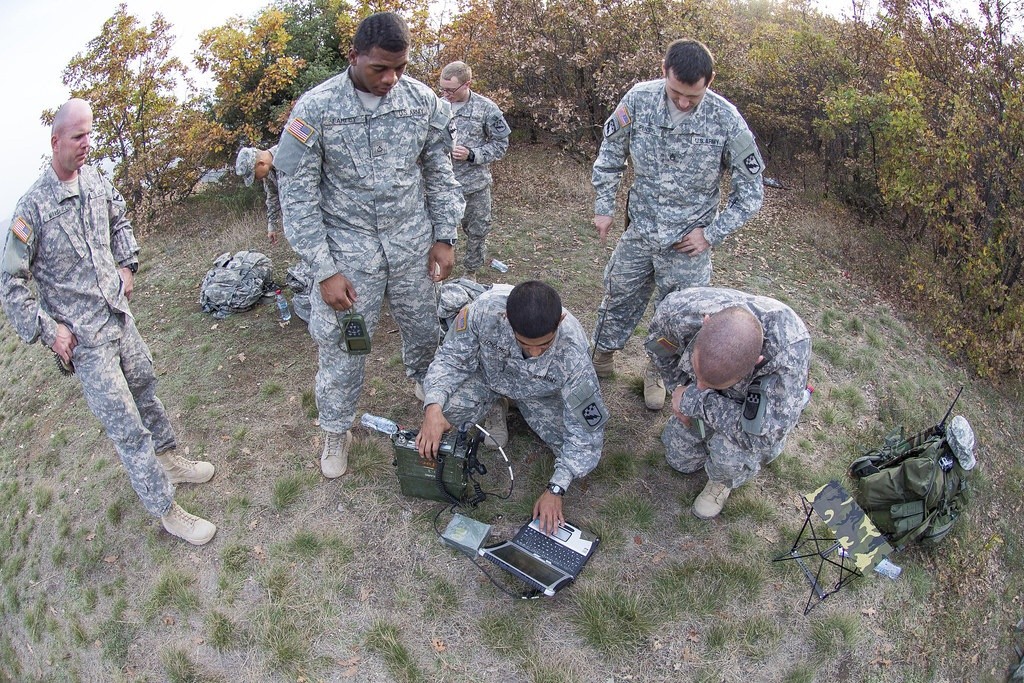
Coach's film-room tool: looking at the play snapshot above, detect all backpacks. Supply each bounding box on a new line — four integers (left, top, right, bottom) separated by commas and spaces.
846, 423, 968, 549
200, 250, 281, 318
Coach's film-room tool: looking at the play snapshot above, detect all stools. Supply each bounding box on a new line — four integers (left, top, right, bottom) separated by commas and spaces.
771, 480, 894, 616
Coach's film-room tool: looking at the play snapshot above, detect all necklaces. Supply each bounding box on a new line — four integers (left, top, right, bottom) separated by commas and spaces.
435, 239, 459, 246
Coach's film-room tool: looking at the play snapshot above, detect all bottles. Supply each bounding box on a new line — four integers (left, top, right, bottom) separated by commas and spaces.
873, 558, 901, 579
802, 386, 814, 407
487, 258, 508, 273
275, 290, 291, 322
360, 413, 402, 435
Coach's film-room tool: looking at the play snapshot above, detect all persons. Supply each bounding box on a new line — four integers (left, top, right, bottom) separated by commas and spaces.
591, 38, 764, 412
273, 14, 468, 478
415, 282, 610, 537
643, 287, 811, 519
432, 61, 511, 293
1, 98, 216, 548
231, 146, 280, 247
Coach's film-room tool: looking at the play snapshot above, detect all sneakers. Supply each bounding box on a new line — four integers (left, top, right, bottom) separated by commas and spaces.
692, 479, 731, 519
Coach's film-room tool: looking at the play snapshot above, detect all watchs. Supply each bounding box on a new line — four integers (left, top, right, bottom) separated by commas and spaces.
469, 150, 473, 161
125, 263, 138, 273
545, 483, 566, 497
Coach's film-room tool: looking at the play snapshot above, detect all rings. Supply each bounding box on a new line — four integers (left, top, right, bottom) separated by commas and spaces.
459, 153, 461, 156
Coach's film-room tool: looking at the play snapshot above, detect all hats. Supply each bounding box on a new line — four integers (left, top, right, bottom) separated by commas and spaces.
946, 415, 976, 470
235, 147, 257, 187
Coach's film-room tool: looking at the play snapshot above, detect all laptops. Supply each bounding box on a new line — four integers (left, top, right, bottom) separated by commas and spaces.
478, 513, 599, 596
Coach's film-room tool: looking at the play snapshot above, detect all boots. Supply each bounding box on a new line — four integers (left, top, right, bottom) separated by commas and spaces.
321, 431, 352, 477
161, 500, 216, 544
643, 358, 666, 409
590, 348, 614, 377
158, 448, 214, 483
484, 397, 509, 448
